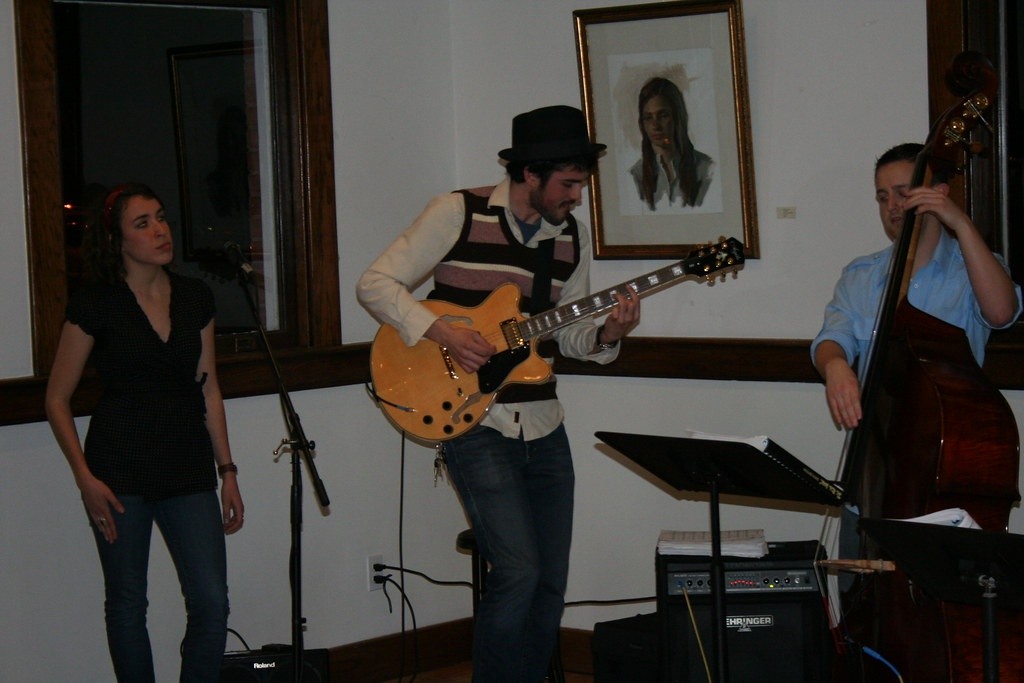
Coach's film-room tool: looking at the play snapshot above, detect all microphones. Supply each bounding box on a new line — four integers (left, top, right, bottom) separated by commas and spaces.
225, 242, 256, 279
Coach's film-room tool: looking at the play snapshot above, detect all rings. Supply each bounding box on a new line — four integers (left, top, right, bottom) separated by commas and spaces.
98, 516, 105, 521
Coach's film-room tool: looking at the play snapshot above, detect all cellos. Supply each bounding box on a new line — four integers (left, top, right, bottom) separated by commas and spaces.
813, 52, 1020, 683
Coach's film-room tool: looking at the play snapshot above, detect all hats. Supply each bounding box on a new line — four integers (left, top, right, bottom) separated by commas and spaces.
496, 106, 607, 164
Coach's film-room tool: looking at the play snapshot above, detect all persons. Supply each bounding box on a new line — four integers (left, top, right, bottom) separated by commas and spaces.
43, 179, 248, 683
356, 104, 645, 683
809, 140, 1023, 654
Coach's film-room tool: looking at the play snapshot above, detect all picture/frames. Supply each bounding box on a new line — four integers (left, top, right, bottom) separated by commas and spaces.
573, 1, 762, 261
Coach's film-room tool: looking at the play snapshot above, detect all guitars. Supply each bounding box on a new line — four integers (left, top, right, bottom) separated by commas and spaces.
370, 235, 745, 448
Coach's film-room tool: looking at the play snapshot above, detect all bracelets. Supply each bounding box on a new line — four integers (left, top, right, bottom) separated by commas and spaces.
217, 462, 239, 476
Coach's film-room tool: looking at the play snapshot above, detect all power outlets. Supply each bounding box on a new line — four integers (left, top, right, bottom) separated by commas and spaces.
366, 553, 386, 592
